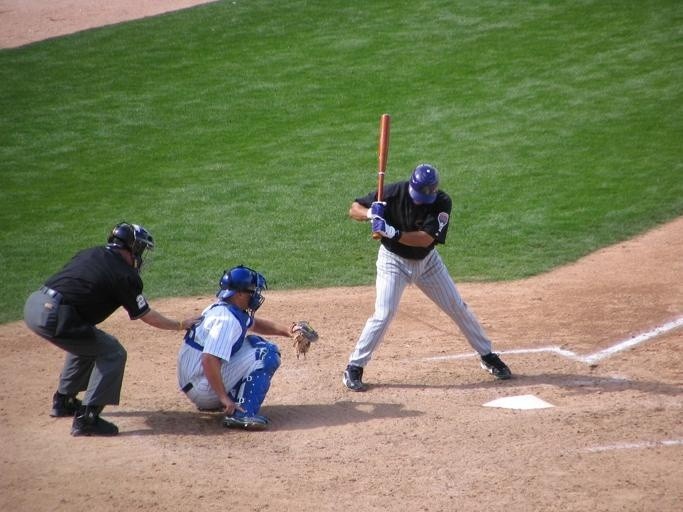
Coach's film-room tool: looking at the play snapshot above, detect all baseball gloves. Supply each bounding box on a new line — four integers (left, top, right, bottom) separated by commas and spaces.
289, 322, 318, 359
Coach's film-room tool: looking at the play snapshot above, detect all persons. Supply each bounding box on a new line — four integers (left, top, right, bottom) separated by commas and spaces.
22, 221, 204, 437
174, 264, 319, 431
340, 163, 513, 392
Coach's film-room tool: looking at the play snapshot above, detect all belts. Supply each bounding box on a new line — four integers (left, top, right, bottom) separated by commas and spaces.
182, 382, 193, 395
40, 285, 70, 308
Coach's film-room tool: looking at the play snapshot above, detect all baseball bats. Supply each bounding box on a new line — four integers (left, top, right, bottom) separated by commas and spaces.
373, 114, 390, 238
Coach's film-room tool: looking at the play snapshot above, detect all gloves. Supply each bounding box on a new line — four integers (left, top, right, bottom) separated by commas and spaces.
365, 201, 387, 219
372, 220, 400, 241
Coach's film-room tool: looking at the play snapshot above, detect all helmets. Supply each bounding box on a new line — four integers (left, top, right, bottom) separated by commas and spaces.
408, 163, 438, 204
106, 222, 154, 270
217, 265, 266, 311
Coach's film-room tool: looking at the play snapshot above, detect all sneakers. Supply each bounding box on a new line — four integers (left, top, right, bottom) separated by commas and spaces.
223, 412, 269, 429
70, 410, 119, 436
481, 353, 510, 379
343, 363, 366, 391
50, 393, 84, 417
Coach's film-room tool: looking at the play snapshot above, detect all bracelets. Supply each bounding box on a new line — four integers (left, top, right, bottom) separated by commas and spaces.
390, 226, 401, 242
177, 320, 183, 330
